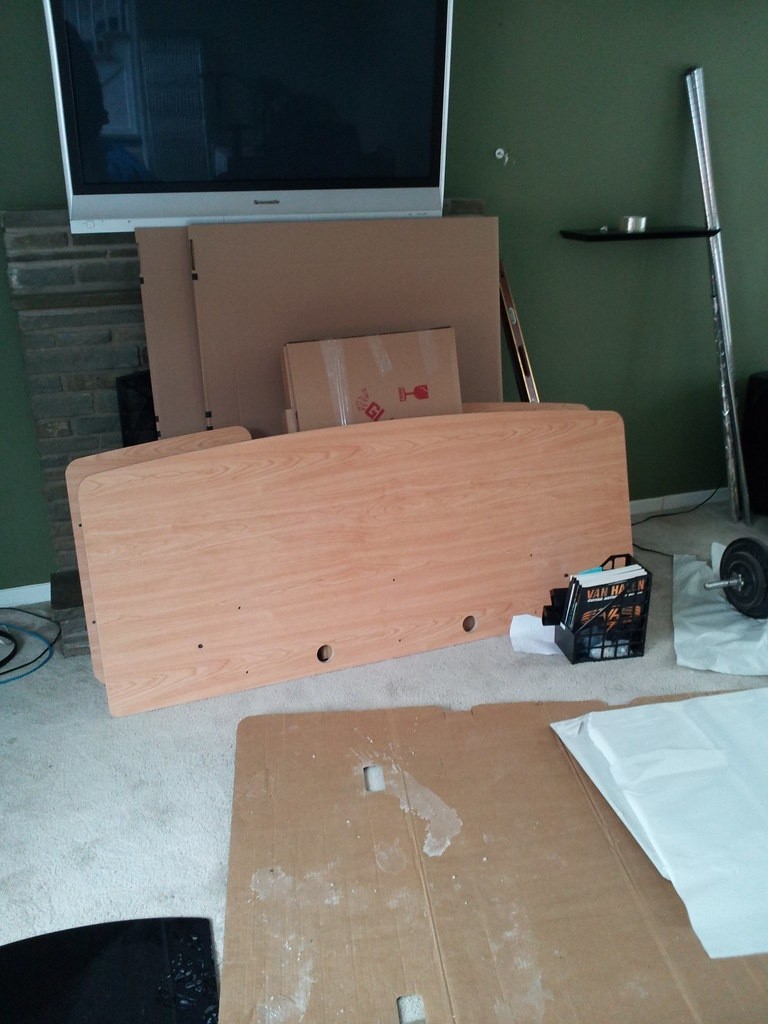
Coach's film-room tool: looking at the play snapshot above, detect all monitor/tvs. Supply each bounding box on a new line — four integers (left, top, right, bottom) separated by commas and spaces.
42, 0, 453, 234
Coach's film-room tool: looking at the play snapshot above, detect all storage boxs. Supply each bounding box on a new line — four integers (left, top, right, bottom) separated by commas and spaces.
280, 328, 464, 437
554, 552, 653, 665
